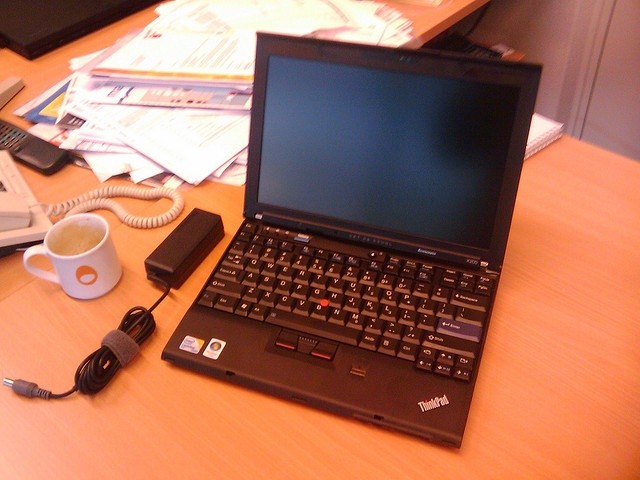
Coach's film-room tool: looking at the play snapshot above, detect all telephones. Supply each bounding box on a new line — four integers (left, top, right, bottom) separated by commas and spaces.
1, 151, 64, 257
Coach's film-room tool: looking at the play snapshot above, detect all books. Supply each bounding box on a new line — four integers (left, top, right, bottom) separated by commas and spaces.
523, 109, 565, 161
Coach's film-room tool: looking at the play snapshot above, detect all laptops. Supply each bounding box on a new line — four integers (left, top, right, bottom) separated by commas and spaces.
161, 31, 544, 450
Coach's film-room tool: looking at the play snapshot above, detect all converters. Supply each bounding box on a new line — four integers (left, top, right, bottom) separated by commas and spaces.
143, 208, 226, 290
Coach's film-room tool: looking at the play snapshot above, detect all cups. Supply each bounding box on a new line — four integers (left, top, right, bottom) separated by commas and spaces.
22, 212, 123, 300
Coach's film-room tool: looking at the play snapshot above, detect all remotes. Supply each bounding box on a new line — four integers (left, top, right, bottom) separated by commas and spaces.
0, 119, 69, 176
